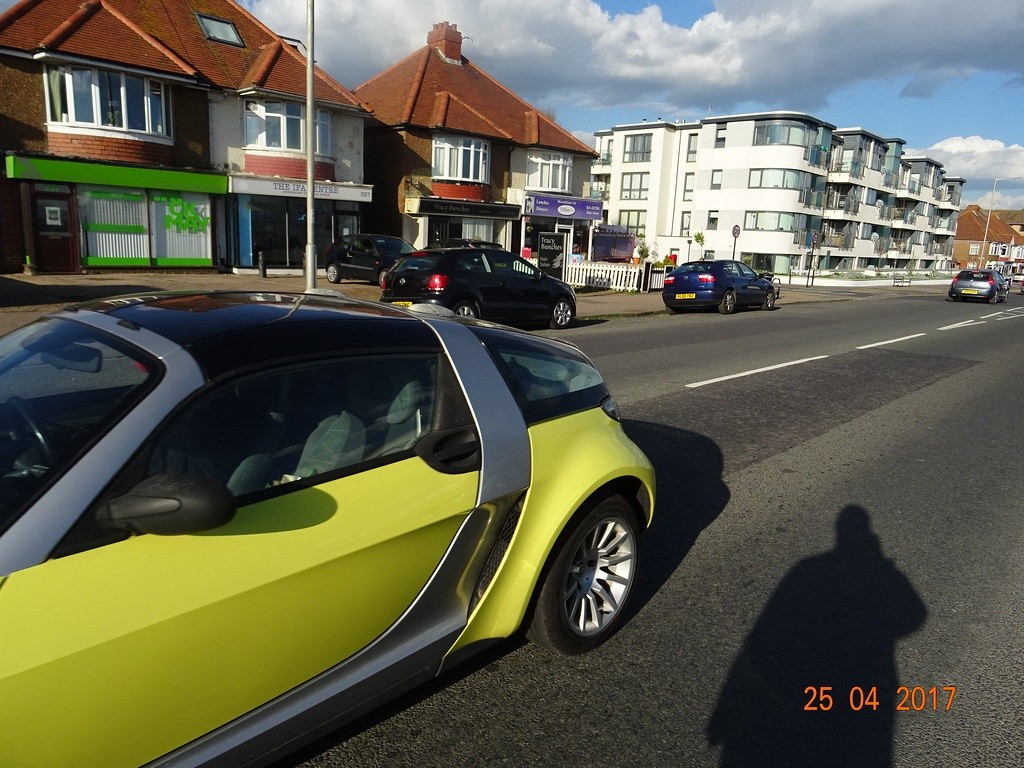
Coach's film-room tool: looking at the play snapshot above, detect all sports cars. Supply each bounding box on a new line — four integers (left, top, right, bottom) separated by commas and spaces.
0, 292, 660, 767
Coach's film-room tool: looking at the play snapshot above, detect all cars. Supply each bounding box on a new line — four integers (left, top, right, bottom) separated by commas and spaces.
947, 267, 1007, 305
423, 236, 511, 274
1000, 271, 1024, 296
324, 233, 419, 289
662, 257, 779, 314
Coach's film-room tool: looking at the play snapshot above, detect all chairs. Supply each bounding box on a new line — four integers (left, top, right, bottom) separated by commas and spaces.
342, 364, 430, 464
183, 364, 290, 460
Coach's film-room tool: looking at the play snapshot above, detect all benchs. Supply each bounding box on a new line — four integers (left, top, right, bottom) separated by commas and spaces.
892, 276, 911, 287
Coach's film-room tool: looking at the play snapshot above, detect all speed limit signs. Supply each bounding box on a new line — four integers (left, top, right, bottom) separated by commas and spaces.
813, 234, 819, 244
733, 224, 741, 238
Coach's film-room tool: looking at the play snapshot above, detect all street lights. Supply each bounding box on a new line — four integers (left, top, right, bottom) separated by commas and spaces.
977, 176, 1022, 269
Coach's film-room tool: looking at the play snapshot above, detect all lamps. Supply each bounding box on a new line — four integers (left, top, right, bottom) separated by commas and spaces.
404, 180, 420, 191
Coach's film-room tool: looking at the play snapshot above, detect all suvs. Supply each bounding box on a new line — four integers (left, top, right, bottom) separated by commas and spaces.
376, 238, 577, 330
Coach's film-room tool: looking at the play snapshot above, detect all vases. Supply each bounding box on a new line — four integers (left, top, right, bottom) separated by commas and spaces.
632, 257, 641, 265
